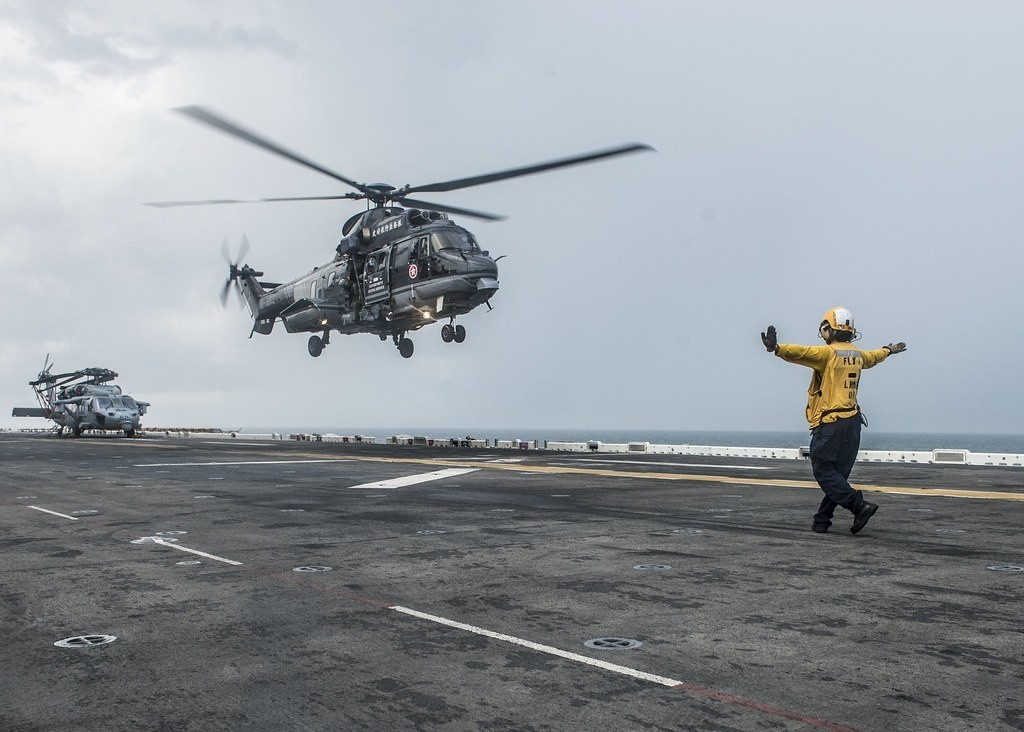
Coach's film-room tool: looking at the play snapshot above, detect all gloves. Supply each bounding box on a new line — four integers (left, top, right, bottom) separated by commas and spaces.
761, 325, 777, 352
882, 342, 907, 356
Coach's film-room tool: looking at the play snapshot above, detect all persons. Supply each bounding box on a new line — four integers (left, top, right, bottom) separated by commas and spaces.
761, 306, 907, 534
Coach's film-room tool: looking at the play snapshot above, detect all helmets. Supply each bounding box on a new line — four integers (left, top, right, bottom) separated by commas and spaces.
818, 306, 856, 345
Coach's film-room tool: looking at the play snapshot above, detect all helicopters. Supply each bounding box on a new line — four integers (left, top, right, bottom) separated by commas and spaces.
13, 353, 150, 438
144, 103, 657, 359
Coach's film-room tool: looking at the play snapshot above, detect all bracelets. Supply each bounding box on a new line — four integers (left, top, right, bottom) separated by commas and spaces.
882, 346, 892, 356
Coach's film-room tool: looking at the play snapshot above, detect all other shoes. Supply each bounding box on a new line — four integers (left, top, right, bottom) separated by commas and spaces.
850, 501, 879, 535
811, 521, 832, 532
